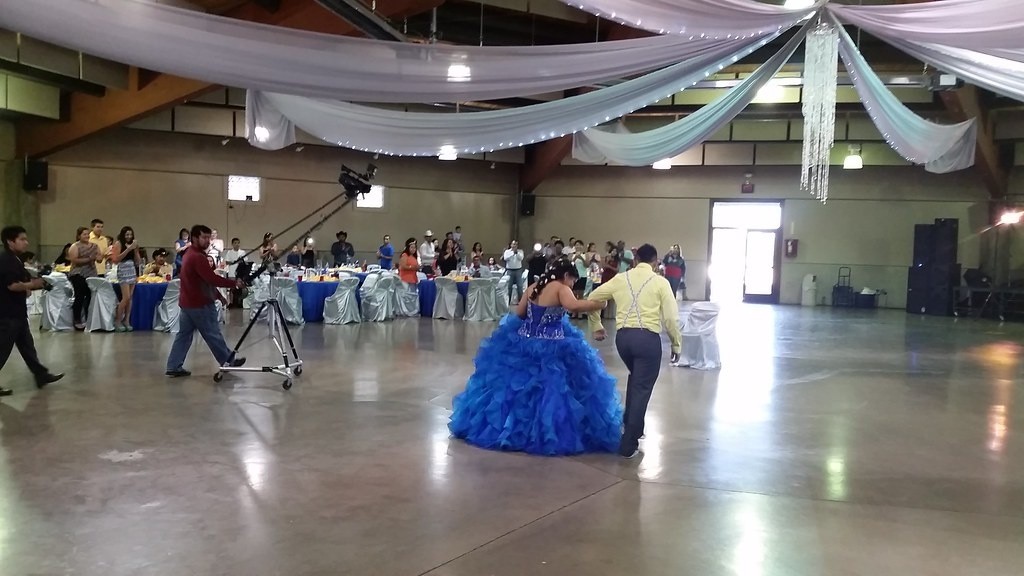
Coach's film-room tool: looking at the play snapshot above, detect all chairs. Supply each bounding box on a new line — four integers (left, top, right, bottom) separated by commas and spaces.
25, 266, 76, 333
152, 279, 181, 333
83, 277, 126, 333
322, 263, 421, 325
432, 268, 529, 322
249, 263, 306, 326
670, 301, 723, 370
213, 269, 229, 325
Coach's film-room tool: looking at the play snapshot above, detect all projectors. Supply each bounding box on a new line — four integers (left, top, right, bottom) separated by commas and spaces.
926, 71, 965, 92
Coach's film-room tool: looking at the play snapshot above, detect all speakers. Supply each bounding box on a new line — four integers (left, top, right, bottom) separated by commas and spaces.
964, 268, 993, 288
26, 161, 48, 191
906, 218, 962, 316
521, 194, 536, 215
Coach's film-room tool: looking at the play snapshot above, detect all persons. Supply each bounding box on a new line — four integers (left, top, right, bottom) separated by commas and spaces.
399, 226, 463, 292
165, 225, 246, 377
587, 244, 682, 457
0, 225, 64, 395
206, 229, 248, 307
288, 235, 316, 269
469, 240, 524, 305
55, 218, 191, 331
447, 258, 624, 456
662, 244, 686, 299
377, 235, 394, 270
22, 251, 36, 267
260, 232, 277, 267
331, 231, 354, 268
526, 236, 637, 320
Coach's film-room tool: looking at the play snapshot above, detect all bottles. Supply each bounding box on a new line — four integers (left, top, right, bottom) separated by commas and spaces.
465, 274, 468, 280
34, 257, 173, 281
284, 258, 367, 282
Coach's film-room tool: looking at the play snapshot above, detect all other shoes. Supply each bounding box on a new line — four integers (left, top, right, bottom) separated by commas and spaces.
623, 449, 639, 458
74, 324, 85, 330
115, 322, 126, 332
34, 372, 64, 389
124, 322, 133, 331
230, 357, 246, 367
570, 314, 576, 319
578, 314, 582, 319
0, 384, 13, 396
165, 366, 191, 377
621, 423, 645, 439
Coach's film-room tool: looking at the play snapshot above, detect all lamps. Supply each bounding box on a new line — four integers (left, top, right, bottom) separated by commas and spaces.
437, 140, 459, 161
445, 44, 473, 83
842, 143, 865, 170
645, 154, 672, 169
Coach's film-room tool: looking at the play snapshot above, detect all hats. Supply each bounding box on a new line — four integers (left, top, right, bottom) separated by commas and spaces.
336, 230, 347, 239
152, 248, 169, 259
425, 230, 435, 237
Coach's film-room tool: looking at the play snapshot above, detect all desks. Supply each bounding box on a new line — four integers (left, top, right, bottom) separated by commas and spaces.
354, 272, 367, 316
296, 281, 340, 323
110, 282, 168, 331
418, 279, 470, 318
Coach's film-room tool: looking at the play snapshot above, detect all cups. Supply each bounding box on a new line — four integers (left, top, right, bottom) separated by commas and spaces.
427, 274, 432, 280
320, 276, 324, 281
433, 275, 437, 281
298, 276, 302, 282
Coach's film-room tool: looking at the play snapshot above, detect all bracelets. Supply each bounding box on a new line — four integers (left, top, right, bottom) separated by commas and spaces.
410, 265, 412, 269
